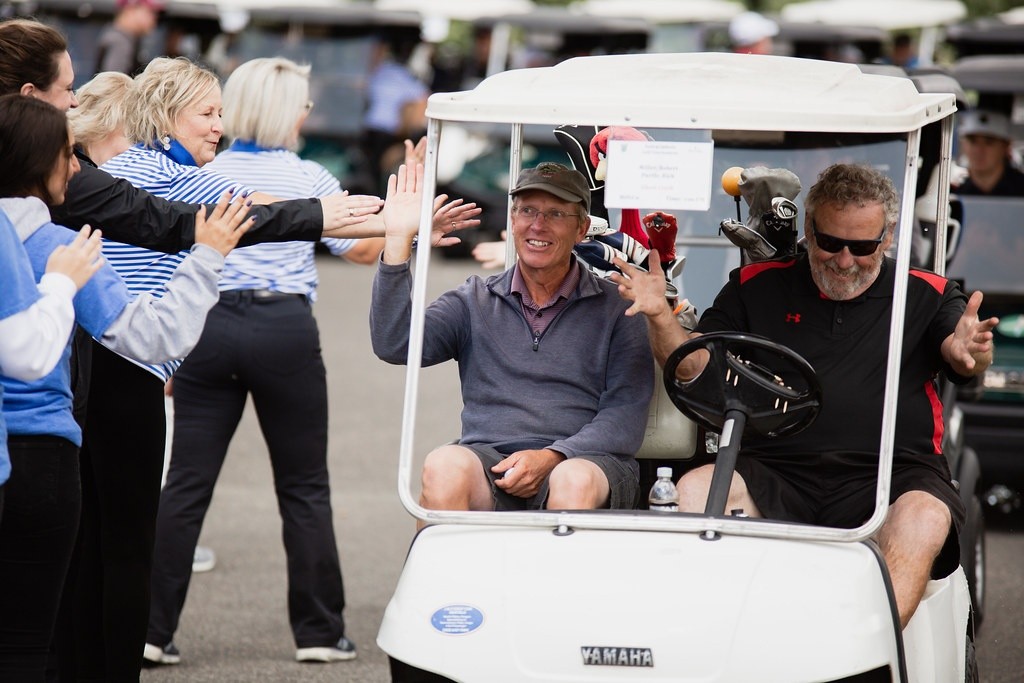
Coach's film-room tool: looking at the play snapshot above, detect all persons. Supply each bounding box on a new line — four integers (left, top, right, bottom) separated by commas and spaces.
0, 21, 481, 683
95, 0, 166, 77
606, 164, 1001, 639
369, 163, 656, 516
951, 110, 1024, 198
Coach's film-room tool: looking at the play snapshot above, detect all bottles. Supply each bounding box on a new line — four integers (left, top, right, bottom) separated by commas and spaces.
648, 466, 680, 512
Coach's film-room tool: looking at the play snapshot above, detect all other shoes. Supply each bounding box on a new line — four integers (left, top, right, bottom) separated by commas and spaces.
191, 544, 217, 572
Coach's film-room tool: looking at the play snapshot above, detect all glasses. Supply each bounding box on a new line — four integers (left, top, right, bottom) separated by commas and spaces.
810, 215, 887, 257
305, 100, 314, 117
513, 204, 580, 221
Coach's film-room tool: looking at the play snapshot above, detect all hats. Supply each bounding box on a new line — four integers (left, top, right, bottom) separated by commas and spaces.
958, 111, 1012, 140
728, 11, 780, 47
119, 0, 168, 11
508, 161, 591, 216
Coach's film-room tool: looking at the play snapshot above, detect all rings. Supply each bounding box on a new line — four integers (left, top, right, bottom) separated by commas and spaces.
350, 209, 355, 217
452, 222, 456, 232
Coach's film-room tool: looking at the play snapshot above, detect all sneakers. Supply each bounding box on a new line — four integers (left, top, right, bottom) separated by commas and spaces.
144, 641, 181, 664
295, 635, 358, 661
